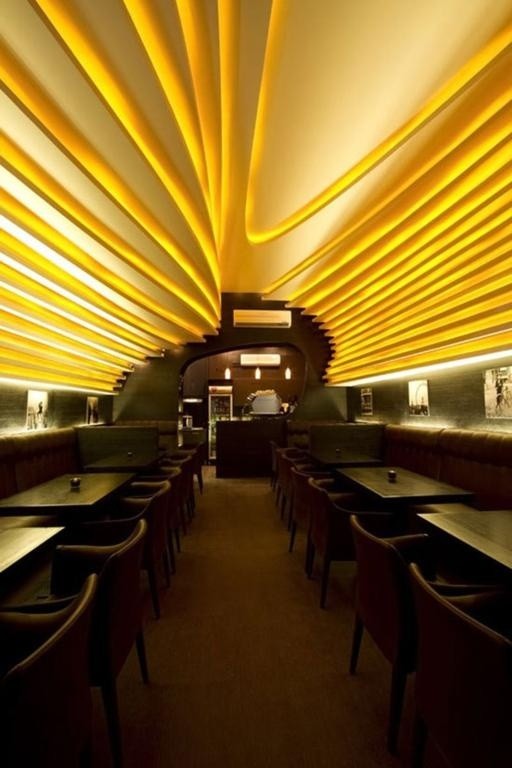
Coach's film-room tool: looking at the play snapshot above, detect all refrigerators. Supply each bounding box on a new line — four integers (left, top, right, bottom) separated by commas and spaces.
207, 385, 234, 465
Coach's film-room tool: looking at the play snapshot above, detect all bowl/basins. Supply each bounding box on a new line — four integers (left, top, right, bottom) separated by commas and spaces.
252, 394, 282, 413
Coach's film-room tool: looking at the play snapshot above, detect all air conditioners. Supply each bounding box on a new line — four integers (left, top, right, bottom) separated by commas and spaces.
232, 307, 293, 329
240, 352, 281, 368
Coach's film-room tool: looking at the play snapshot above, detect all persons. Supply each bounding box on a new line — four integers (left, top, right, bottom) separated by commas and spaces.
495, 374, 512, 414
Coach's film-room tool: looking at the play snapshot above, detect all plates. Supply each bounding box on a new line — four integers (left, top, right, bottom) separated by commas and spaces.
249, 412, 282, 417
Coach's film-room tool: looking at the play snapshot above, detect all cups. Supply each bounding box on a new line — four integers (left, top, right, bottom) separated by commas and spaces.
183, 415, 192, 431
128, 451, 133, 457
69, 477, 80, 489
335, 449, 340, 454
387, 470, 396, 479
